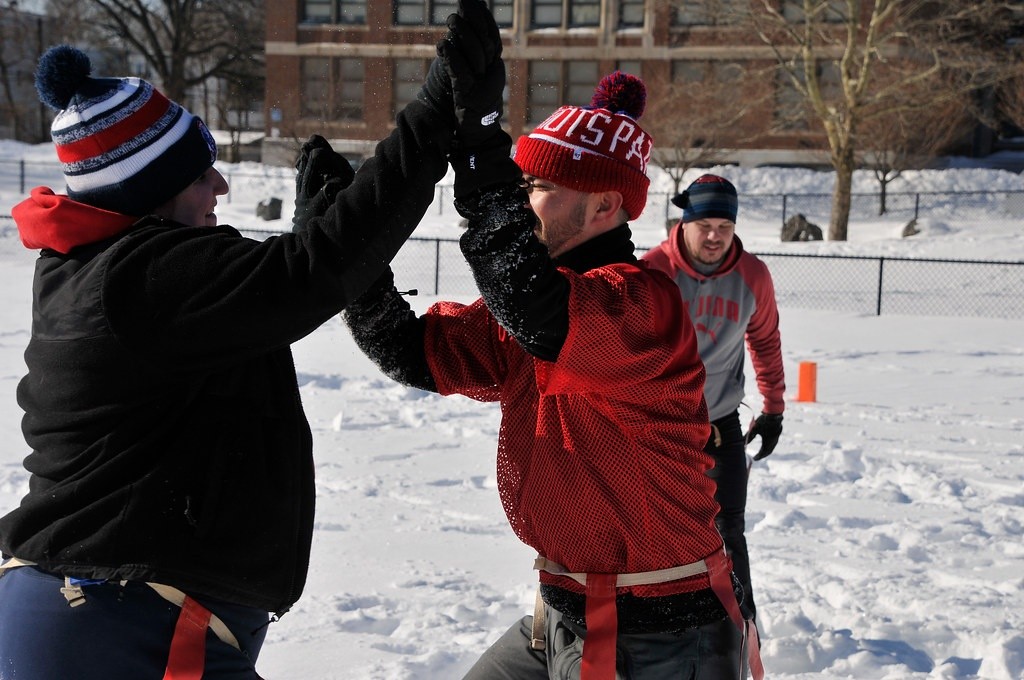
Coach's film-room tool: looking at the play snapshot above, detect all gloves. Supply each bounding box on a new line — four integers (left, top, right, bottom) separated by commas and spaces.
416, 0, 505, 144
742, 412, 783, 461
289, 134, 355, 234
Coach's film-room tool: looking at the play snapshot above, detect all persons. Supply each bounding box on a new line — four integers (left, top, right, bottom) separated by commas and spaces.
0, 0, 765, 680
638, 173, 786, 650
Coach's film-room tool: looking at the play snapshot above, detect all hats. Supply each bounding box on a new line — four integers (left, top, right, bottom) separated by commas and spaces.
32, 44, 218, 219
671, 173, 738, 224
514, 70, 653, 221
